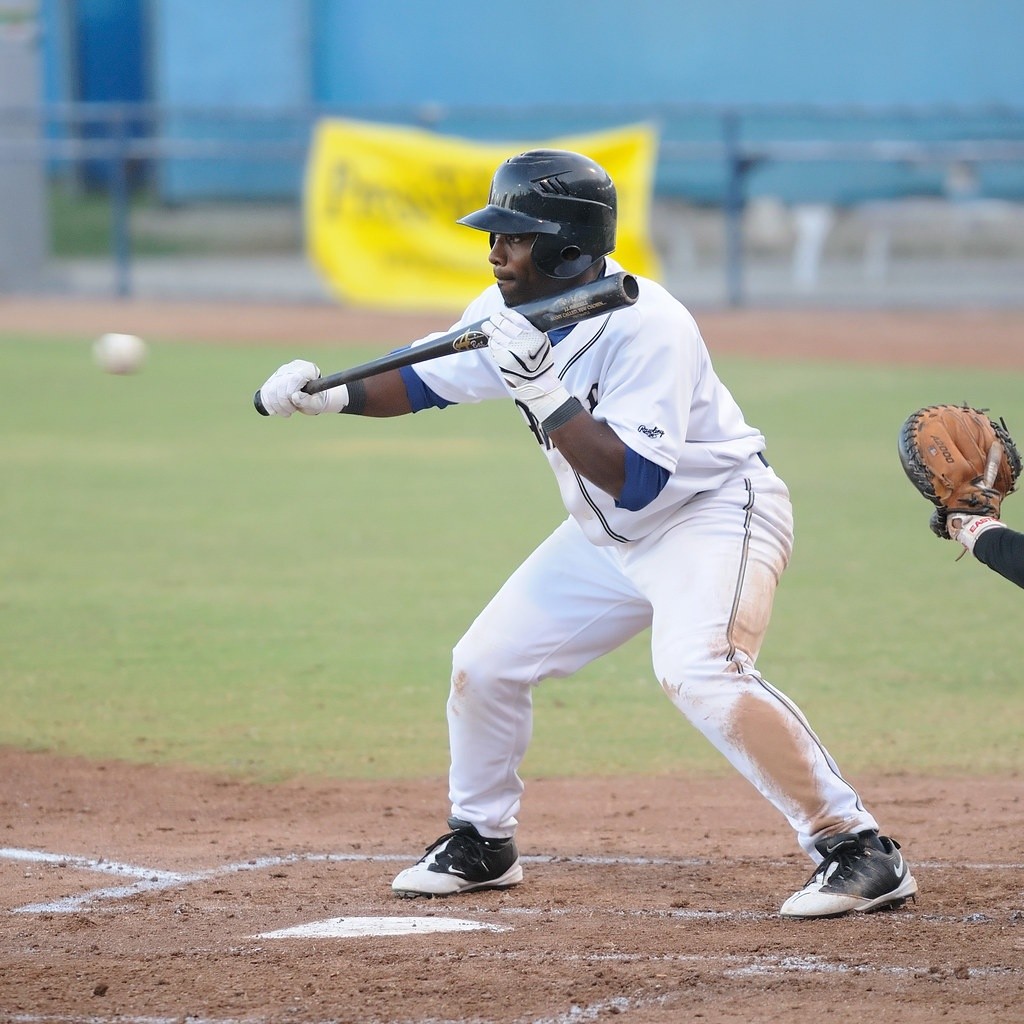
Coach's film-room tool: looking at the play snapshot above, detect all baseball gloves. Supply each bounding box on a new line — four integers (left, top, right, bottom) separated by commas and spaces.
896, 400, 1024, 562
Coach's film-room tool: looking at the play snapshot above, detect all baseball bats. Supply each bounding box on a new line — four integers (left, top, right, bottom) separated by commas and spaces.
254, 271, 642, 418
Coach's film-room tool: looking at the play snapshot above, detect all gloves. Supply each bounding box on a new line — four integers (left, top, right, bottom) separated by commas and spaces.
480, 308, 584, 425
259, 359, 350, 418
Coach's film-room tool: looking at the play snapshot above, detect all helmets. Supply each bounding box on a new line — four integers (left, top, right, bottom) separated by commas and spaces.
456, 148, 618, 281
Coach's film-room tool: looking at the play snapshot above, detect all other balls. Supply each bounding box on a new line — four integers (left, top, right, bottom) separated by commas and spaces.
95, 332, 142, 374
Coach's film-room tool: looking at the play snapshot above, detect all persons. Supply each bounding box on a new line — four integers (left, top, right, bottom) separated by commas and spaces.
260, 151, 917, 915
929, 506, 1024, 590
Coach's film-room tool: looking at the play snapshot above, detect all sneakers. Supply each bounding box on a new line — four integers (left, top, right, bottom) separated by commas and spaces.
391, 816, 525, 899
778, 831, 918, 921
